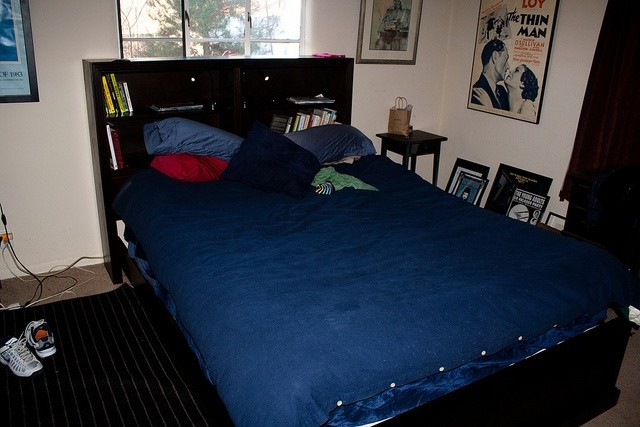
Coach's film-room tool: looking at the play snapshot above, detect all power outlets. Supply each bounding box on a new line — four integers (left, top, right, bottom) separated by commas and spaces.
0, 230, 14, 249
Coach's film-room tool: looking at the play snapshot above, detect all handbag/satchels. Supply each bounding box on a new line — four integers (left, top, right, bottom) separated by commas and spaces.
388, 97, 411, 136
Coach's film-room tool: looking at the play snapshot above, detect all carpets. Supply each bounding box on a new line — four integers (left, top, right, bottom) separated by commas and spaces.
0, 282, 234, 427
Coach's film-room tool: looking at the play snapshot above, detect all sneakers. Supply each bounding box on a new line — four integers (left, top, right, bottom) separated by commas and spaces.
25, 319, 56, 358
0, 336, 44, 378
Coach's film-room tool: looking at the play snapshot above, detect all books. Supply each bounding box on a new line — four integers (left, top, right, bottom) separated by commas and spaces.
271, 108, 338, 134
286, 94, 336, 105
101, 73, 133, 114
149, 101, 204, 112
105, 122, 124, 172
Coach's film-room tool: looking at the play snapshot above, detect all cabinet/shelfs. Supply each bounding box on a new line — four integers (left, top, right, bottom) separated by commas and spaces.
82, 58, 354, 284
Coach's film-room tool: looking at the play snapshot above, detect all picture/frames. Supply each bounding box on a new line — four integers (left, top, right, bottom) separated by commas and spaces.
482, 162, 553, 219
467, 0, 559, 125
451, 171, 489, 206
0, 0, 39, 103
445, 158, 489, 194
502, 185, 551, 226
356, 0, 423, 65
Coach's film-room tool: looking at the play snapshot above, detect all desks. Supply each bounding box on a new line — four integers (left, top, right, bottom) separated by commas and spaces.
377, 130, 447, 187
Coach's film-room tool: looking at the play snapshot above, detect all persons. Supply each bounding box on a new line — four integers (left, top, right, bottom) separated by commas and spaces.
471, 40, 510, 111
461, 187, 471, 203
494, 16, 504, 38
486, 14, 496, 39
473, 64, 539, 118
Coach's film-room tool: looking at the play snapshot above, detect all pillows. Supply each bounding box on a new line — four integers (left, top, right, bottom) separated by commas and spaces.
144, 117, 244, 163
219, 121, 320, 199
282, 125, 375, 164
150, 154, 229, 182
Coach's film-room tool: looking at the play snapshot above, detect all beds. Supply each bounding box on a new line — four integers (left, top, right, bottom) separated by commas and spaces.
111, 155, 640, 427
